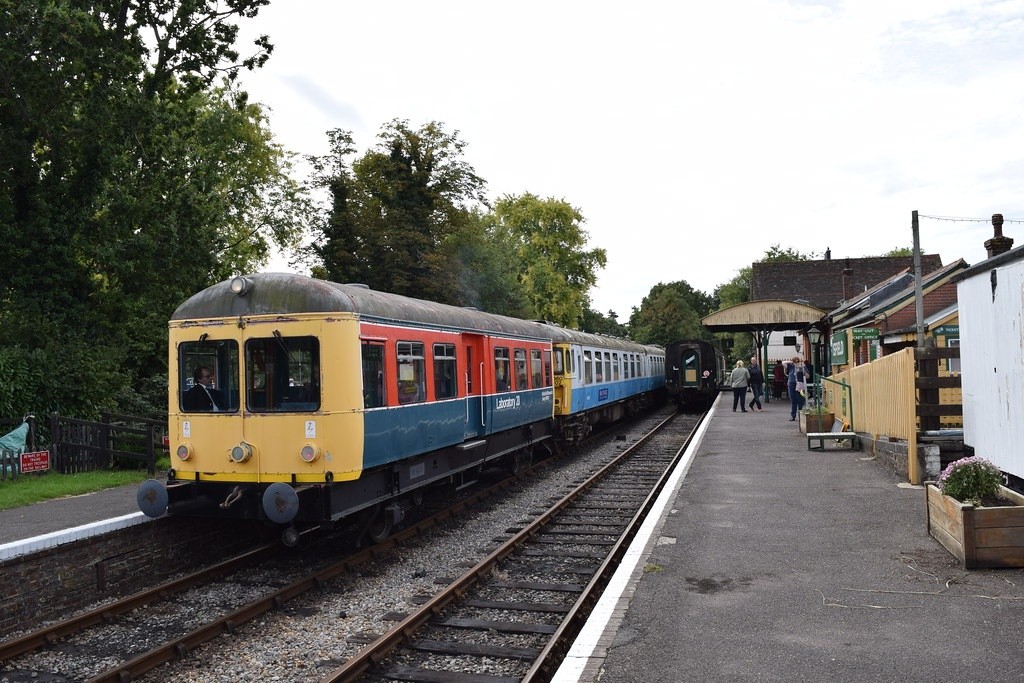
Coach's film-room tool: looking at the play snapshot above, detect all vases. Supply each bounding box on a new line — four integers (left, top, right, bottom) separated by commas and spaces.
922, 479, 1024, 568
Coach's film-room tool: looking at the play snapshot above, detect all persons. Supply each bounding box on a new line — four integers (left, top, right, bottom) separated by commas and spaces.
289, 378, 304, 387
254, 376, 260, 389
188, 366, 226, 412
730, 357, 813, 421
496, 369, 527, 392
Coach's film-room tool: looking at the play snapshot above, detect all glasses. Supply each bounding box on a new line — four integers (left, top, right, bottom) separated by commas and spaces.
202, 375, 212, 379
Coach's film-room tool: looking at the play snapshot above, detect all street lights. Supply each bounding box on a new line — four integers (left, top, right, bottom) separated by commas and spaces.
807, 324, 823, 447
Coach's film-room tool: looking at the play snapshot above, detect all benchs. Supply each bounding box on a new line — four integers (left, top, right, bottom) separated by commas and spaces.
806, 432, 857, 451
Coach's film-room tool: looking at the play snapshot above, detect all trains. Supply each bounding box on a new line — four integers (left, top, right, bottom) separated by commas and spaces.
137, 272, 666, 546
664, 339, 726, 411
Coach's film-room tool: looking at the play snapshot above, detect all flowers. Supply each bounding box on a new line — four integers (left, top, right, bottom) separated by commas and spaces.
936, 454, 1003, 505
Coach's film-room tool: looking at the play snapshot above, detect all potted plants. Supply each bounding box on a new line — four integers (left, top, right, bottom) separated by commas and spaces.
800, 407, 836, 435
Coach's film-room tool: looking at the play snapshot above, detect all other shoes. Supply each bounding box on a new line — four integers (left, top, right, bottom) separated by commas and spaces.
741, 410, 747, 412
758, 409, 763, 412
733, 409, 736, 412
789, 417, 795, 421
749, 404, 754, 411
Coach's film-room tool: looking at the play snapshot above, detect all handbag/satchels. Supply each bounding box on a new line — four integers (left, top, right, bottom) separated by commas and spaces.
795, 382, 805, 391
